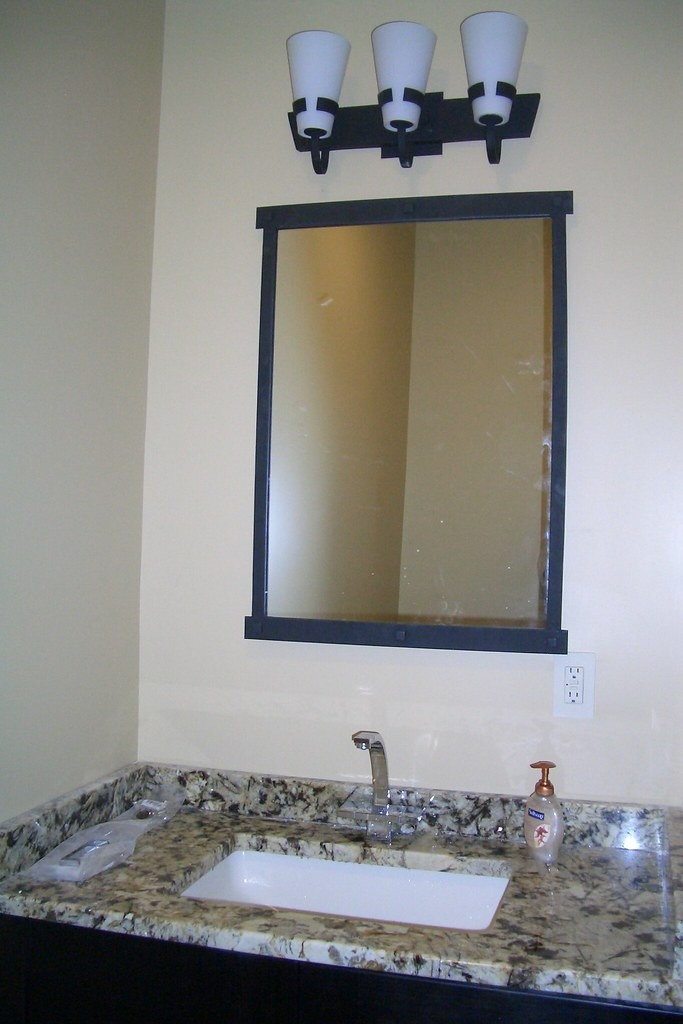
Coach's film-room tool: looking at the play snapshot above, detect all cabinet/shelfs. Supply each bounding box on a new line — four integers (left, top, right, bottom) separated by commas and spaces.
0, 913, 683, 1024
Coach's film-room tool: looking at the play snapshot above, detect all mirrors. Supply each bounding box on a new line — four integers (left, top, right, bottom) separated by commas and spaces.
245, 190, 573, 655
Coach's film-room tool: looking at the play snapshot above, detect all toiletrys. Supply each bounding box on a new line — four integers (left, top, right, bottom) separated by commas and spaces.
523, 761, 564, 863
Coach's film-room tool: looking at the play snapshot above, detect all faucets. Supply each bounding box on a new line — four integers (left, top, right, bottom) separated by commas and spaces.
352, 728, 389, 806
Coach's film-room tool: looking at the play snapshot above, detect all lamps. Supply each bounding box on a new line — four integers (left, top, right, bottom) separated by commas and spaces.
286, 29, 350, 173
460, 12, 528, 165
371, 21, 438, 166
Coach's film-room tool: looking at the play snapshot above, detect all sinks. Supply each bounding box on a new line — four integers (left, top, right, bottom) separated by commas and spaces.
166, 833, 514, 932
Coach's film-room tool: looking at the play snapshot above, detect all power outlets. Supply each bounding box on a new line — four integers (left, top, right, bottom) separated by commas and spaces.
551, 651, 596, 721
